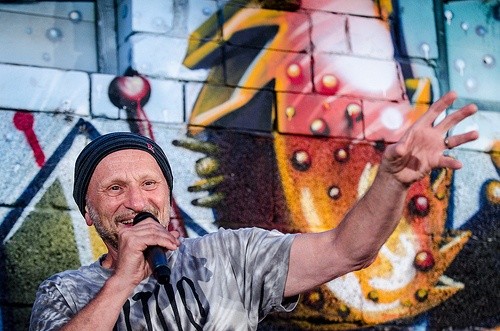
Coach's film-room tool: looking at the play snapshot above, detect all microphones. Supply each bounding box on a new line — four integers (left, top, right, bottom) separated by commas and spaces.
133, 212, 171, 285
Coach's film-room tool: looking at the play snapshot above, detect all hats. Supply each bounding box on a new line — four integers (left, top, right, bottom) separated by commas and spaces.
73, 132, 173, 217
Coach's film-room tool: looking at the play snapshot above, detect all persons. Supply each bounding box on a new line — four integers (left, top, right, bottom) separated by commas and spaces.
29, 90, 478, 331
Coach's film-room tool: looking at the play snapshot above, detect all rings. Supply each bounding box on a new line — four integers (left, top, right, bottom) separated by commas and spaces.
444, 135, 454, 150
